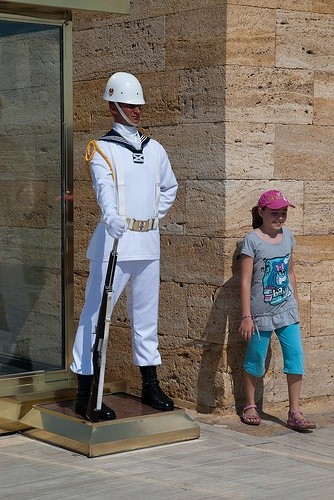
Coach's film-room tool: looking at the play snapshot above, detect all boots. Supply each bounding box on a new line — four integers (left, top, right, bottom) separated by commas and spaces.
139, 366, 174, 411
75, 375, 116, 420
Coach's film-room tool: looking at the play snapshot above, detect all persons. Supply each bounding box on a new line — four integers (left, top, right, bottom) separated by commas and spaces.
70, 71, 179, 422
239, 190, 317, 430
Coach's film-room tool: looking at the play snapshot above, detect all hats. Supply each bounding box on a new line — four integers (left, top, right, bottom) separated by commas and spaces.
258, 190, 295, 210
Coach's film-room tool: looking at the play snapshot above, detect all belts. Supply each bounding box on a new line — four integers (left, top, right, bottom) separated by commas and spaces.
125, 218, 157, 231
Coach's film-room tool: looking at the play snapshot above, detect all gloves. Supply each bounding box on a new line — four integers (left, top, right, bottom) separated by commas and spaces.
106, 218, 128, 238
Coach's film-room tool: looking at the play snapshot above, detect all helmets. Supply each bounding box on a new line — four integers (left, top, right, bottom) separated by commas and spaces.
103, 72, 145, 104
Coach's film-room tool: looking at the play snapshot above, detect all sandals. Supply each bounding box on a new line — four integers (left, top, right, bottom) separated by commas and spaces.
287, 409, 316, 430
241, 405, 261, 425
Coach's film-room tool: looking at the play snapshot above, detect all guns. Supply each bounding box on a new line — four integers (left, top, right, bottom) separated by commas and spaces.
88, 237, 119, 412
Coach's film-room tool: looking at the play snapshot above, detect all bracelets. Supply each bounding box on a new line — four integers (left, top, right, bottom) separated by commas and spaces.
240, 315, 251, 319
